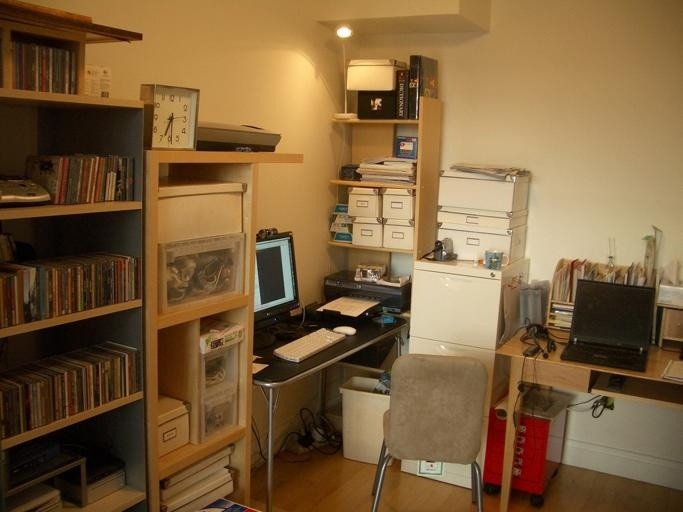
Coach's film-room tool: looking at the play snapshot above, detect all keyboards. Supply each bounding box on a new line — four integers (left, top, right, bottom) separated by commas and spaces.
273, 327, 346, 363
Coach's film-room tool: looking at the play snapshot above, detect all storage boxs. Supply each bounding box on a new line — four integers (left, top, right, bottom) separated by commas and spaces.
158, 393, 190, 457
201, 343, 238, 392
347, 57, 406, 92
436, 168, 530, 266
346, 188, 414, 248
152, 177, 247, 239
158, 232, 246, 314
200, 392, 238, 444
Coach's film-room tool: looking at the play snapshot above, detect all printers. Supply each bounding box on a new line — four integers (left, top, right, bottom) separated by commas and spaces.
302, 270, 411, 324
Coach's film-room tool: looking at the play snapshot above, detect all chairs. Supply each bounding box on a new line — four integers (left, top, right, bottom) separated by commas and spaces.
371, 355, 488, 511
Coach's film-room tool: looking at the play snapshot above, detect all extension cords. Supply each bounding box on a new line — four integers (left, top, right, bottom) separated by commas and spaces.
296, 438, 326, 452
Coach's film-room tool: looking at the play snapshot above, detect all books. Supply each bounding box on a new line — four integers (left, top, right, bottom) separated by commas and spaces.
356, 157, 417, 186
0, 231, 142, 329
0, 340, 140, 441
26, 152, 134, 205
553, 236, 655, 331
6, 463, 126, 512
12, 40, 76, 95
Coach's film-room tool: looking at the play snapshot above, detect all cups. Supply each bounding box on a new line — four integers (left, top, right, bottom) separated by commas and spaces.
484, 249, 509, 270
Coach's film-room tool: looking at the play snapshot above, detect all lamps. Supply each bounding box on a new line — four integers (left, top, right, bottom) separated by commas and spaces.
334, 23, 356, 120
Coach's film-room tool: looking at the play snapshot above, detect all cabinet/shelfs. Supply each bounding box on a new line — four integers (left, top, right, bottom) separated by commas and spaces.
484, 380, 573, 507
1, 1, 146, 512
146, 150, 304, 512
329, 97, 445, 315
400, 255, 529, 491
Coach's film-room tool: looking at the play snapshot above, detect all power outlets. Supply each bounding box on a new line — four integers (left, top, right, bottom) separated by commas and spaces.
602, 395, 615, 410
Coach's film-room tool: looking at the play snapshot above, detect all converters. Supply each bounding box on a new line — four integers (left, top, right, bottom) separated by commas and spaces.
298, 435, 313, 446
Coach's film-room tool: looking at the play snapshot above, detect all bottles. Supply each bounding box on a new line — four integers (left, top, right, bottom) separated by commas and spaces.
432, 240, 441, 262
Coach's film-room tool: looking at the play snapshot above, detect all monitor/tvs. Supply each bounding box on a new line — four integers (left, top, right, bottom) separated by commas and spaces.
254, 235, 299, 330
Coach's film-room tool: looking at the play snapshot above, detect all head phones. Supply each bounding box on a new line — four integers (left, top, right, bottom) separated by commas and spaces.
528, 324, 549, 339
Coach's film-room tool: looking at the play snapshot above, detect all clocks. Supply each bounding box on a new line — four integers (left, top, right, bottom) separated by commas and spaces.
141, 82, 199, 150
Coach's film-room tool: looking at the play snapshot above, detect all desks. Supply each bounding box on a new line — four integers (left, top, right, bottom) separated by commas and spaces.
496, 325, 683, 512
251, 312, 407, 512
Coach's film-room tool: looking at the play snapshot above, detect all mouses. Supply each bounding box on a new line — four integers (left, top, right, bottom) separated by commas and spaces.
332, 325, 357, 336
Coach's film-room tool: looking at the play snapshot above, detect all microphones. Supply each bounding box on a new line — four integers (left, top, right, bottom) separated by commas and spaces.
534, 340, 548, 359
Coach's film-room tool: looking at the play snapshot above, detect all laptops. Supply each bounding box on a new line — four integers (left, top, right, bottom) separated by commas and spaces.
560, 278, 656, 373
